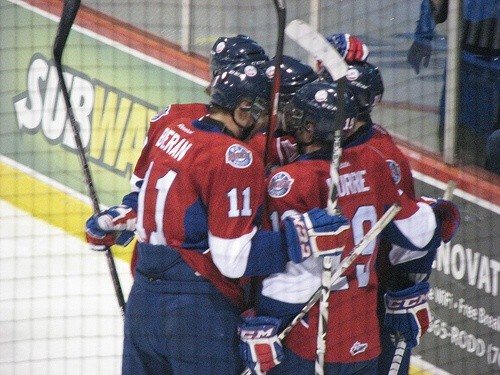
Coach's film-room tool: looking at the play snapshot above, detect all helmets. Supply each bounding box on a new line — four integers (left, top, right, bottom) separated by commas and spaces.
261, 55, 319, 101
289, 81, 357, 145
210, 35, 271, 81
210, 62, 272, 122
317, 32, 370, 72
319, 60, 384, 119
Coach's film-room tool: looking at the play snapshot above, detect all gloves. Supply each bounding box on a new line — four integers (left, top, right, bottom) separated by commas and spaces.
237, 307, 284, 375
408, 41, 433, 74
84, 204, 137, 252
435, 198, 461, 244
281, 207, 351, 265
383, 282, 432, 350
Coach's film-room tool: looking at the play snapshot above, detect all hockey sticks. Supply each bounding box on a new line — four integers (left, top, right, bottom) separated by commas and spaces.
387, 177, 458, 375
51, 0, 127, 329
236, 200, 404, 375
283, 17, 352, 375
262, 0, 288, 169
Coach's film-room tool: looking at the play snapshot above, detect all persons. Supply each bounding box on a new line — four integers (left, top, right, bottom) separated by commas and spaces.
407, 0, 500, 170
84, 34, 461, 375
